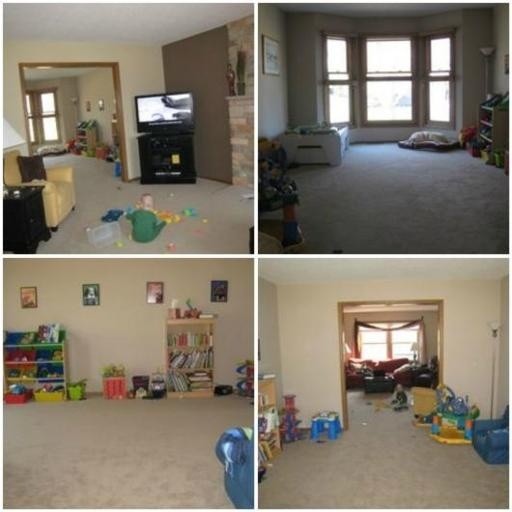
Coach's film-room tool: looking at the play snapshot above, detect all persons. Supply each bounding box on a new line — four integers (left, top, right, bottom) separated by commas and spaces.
83, 286, 99, 305
126, 193, 167, 244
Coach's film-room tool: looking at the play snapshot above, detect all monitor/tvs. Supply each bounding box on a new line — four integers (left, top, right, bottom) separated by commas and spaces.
134, 89, 195, 135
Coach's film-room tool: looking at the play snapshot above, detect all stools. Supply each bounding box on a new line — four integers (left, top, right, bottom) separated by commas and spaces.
311, 411, 341, 440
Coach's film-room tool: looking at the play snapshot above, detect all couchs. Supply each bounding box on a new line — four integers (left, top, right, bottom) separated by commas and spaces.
346, 358, 411, 388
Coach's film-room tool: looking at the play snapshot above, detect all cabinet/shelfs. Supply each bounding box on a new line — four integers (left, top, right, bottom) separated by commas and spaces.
74, 126, 96, 150
4, 328, 68, 399
478, 94, 509, 153
258, 378, 282, 460
165, 319, 216, 401
137, 132, 196, 185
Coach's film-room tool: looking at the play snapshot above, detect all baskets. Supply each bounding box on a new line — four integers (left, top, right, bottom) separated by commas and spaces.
5, 383, 85, 402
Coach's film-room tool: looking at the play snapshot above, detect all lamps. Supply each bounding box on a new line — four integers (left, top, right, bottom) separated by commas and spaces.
2, 118, 25, 195
410, 342, 421, 363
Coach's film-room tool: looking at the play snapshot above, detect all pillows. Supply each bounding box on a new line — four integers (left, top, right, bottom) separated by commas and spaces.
350, 363, 367, 374
18, 155, 47, 183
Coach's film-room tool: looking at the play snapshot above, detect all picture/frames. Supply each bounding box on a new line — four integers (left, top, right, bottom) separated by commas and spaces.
210, 279, 228, 303
145, 281, 163, 304
20, 287, 37, 309
81, 283, 100, 307
262, 34, 282, 75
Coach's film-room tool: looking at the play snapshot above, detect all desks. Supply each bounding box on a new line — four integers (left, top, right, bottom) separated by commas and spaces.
3, 183, 51, 254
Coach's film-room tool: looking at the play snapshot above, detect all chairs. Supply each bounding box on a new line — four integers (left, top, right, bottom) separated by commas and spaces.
4, 149, 75, 232
474, 404, 509, 465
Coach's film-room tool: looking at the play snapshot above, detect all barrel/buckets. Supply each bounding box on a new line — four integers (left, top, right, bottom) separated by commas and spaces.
132, 375, 148, 394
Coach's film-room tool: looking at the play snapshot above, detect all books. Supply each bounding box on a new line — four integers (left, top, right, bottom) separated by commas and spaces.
167, 349, 214, 368
167, 370, 213, 391
167, 333, 214, 346
